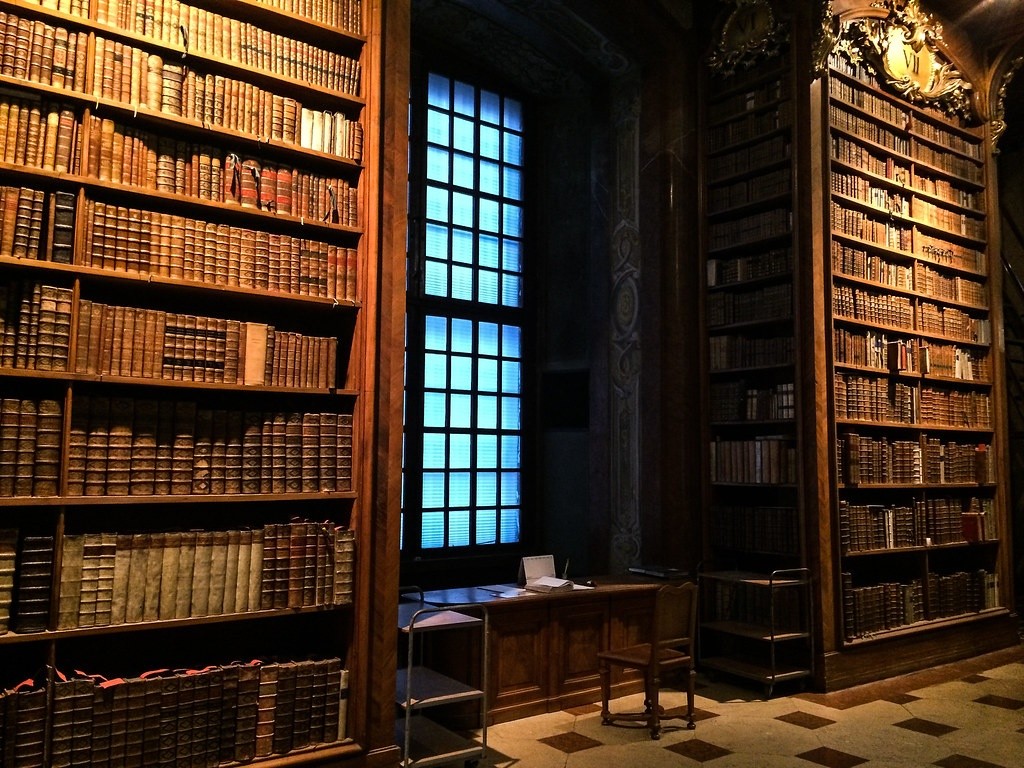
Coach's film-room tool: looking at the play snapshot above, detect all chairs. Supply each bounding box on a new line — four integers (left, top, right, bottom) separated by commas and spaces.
598, 583, 699, 740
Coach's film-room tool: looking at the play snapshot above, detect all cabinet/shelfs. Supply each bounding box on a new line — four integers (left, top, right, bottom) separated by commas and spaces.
405, 568, 697, 729
694, 558, 818, 699
696, 1, 1024, 682
397, 584, 489, 768
0, 0, 405, 768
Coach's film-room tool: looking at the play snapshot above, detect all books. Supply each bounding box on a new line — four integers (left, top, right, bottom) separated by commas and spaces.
705, 53, 984, 183
839, 496, 998, 551
705, 240, 989, 342
714, 579, 802, 633
0, 279, 338, 389
0, 12, 88, 177
0, 186, 76, 266
703, 435, 799, 487
523, 576, 596, 594
841, 570, 999, 639
711, 503, 802, 559
0, 396, 352, 495
87, 52, 362, 228
0, 657, 350, 768
837, 432, 993, 486
704, 168, 986, 274
0, 521, 356, 634
23, 0, 362, 97
707, 329, 992, 428
80, 198, 357, 302
